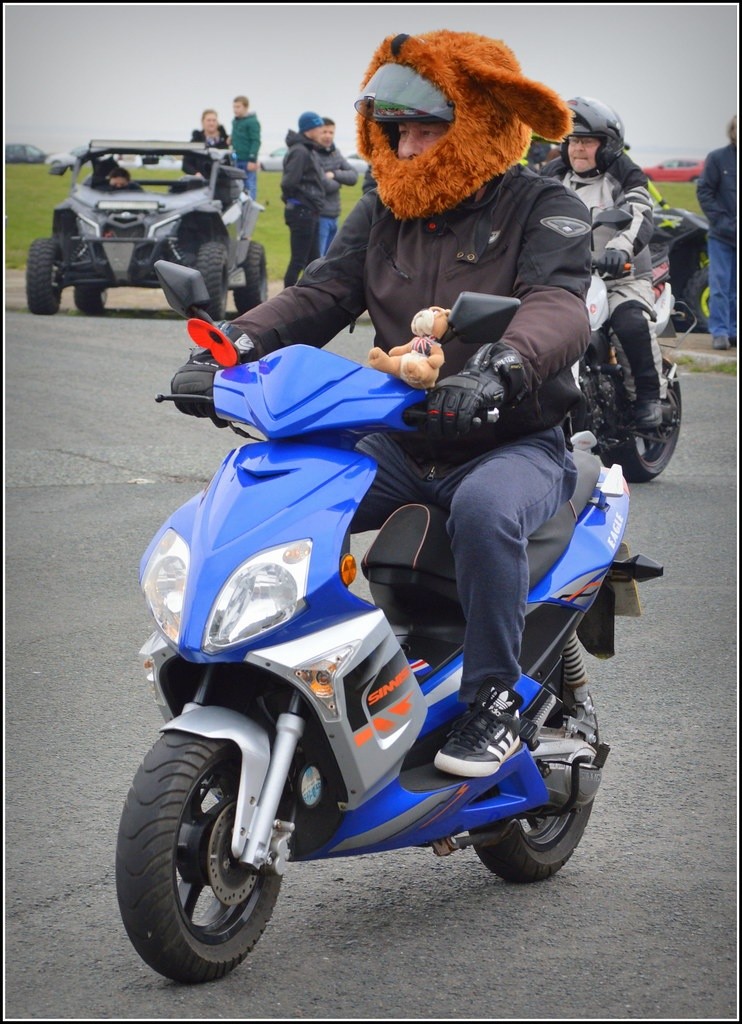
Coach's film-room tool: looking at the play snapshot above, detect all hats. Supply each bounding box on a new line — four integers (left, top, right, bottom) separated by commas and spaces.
298, 111, 324, 132
357, 33, 573, 220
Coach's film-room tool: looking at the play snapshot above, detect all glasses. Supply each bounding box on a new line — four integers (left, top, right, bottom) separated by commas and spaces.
567, 136, 603, 147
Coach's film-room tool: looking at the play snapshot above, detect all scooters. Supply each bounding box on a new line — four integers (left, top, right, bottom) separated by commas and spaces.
576, 209, 682, 483
114, 260, 664, 985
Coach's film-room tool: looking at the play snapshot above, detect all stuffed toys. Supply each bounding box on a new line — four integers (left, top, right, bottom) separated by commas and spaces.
368, 307, 452, 388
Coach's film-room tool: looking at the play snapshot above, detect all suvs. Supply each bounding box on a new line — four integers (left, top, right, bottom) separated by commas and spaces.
26, 139, 269, 321
648, 206, 709, 330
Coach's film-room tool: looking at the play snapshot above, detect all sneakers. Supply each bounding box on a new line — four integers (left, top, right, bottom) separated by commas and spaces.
634, 396, 663, 430
434, 675, 524, 777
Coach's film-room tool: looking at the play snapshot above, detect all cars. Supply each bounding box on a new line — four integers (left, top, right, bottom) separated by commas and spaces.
141, 153, 183, 170
346, 152, 368, 173
44, 144, 142, 168
5, 144, 45, 164
641, 158, 705, 183
259, 146, 287, 171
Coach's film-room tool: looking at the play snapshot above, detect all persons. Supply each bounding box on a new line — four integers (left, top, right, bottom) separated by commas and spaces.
524, 136, 561, 173
172, 30, 591, 777
182, 110, 233, 211
695, 115, 737, 352
96, 168, 144, 192
538, 96, 668, 428
280, 112, 378, 289
226, 95, 261, 201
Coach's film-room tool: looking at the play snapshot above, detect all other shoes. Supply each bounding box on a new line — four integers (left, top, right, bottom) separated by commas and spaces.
712, 333, 736, 352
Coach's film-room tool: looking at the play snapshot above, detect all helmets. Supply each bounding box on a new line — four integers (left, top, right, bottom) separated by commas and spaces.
559, 97, 624, 177
373, 62, 455, 155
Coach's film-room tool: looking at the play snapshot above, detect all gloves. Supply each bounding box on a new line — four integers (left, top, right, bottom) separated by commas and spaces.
597, 248, 628, 278
170, 320, 256, 429
420, 342, 524, 445
